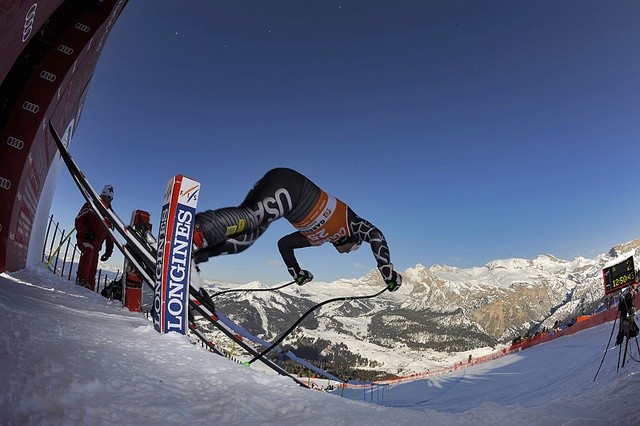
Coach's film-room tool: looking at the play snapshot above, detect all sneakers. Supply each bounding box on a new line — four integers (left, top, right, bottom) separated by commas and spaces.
150, 236, 201, 292
76, 278, 92, 290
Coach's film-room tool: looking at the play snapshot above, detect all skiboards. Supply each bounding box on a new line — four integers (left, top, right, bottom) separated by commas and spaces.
49, 119, 390, 389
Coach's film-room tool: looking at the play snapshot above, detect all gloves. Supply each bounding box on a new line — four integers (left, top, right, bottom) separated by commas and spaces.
100, 253, 108, 260
288, 263, 313, 286
85, 231, 96, 241
378, 263, 402, 292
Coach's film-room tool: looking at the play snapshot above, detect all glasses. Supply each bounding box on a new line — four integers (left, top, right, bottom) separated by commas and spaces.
106, 194, 113, 200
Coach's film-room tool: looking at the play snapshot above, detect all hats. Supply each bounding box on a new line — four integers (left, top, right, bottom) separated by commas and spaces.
347, 236, 362, 253
101, 185, 114, 196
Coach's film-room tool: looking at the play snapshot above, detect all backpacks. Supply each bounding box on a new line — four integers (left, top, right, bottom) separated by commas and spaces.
101, 279, 122, 301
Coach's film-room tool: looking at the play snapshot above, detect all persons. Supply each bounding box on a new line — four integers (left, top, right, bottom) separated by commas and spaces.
192, 167, 402, 311
74, 184, 115, 289
